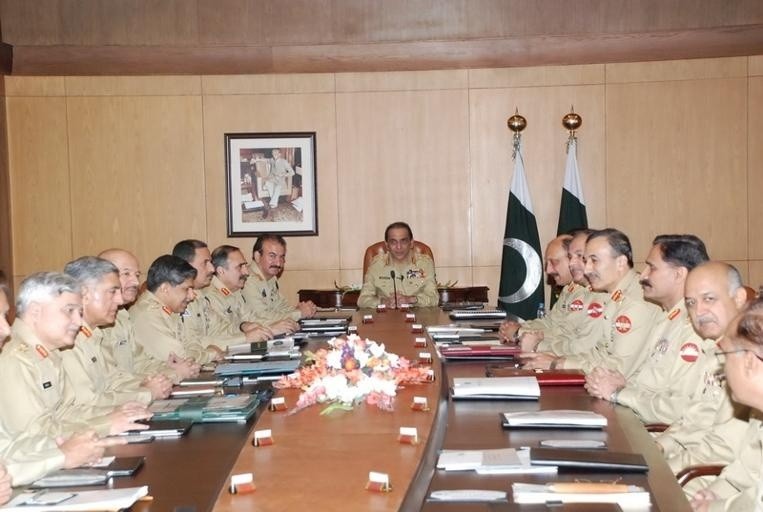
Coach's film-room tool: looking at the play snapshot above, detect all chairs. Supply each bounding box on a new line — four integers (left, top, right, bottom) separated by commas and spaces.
364, 241, 435, 284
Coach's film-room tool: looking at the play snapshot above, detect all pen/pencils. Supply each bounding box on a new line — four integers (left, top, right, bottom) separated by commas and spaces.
138, 496, 154, 501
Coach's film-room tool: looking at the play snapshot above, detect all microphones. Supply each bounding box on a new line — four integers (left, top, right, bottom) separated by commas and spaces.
390, 270, 398, 310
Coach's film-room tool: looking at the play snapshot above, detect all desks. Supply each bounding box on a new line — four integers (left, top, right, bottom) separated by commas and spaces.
2, 304, 693, 512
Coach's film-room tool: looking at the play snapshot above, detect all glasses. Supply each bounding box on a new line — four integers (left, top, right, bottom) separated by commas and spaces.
714, 348, 763, 364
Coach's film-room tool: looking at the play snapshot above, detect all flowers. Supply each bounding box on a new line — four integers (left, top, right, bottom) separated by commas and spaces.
272, 333, 428, 417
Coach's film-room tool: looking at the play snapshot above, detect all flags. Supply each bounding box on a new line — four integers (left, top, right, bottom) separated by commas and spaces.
496, 149, 546, 318
558, 142, 590, 237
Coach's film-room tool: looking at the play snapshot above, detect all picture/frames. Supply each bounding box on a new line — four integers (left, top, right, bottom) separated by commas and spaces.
224, 132, 318, 237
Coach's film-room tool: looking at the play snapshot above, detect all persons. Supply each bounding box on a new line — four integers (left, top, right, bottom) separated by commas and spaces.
249, 149, 295, 220
356, 222, 439, 309
499, 230, 763, 512
1, 235, 314, 504
